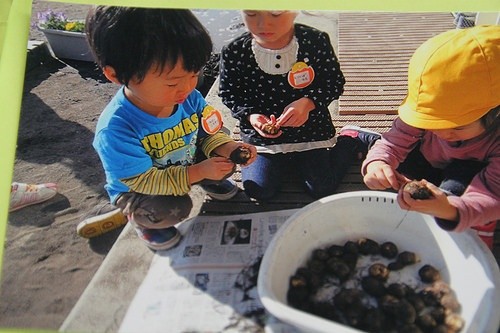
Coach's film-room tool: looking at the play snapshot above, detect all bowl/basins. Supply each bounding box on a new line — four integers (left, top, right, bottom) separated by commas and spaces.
255, 190, 500, 332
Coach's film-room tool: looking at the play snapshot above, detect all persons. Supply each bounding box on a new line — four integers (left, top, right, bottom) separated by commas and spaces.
84, 5, 257, 251
219, 10, 381, 206
361, 24, 500, 251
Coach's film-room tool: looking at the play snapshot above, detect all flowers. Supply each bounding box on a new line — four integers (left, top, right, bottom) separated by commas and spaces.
30, 8, 85, 33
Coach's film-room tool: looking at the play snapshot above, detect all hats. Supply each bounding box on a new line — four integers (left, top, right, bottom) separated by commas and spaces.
398, 25, 500, 130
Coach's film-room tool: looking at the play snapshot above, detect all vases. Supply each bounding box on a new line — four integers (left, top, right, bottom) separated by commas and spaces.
36, 20, 95, 61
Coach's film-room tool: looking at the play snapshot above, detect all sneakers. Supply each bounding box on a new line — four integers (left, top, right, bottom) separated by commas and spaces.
127, 213, 181, 251
198, 178, 237, 199
8, 182, 58, 212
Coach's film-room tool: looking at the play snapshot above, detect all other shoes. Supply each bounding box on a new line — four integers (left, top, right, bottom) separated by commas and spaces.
336, 125, 382, 152
76, 201, 128, 238
